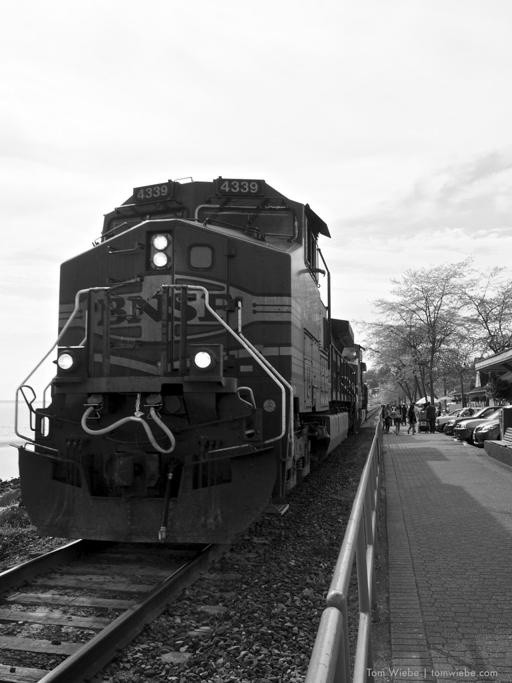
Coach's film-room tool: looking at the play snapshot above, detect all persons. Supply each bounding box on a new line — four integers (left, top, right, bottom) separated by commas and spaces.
437, 401, 442, 416
422, 402, 427, 410
381, 402, 421, 435
424, 400, 437, 434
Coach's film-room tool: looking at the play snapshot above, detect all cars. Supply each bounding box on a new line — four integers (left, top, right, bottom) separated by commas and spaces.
433, 404, 511, 448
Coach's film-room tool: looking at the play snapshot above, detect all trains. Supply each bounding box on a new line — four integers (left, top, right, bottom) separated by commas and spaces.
11, 175, 369, 548
